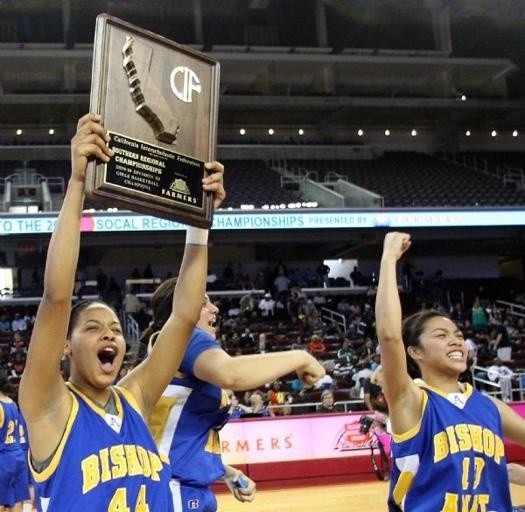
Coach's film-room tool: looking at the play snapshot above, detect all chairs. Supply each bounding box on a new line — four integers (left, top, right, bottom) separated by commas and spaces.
216, 150, 525, 209
0, 158, 71, 196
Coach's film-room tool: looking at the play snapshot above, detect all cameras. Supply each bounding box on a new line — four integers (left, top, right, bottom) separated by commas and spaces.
358, 416, 373, 433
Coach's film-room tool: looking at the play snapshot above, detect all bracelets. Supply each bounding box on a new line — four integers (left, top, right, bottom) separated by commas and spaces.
186, 226, 208, 246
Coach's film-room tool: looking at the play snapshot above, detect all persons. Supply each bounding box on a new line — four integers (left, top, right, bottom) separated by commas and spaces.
139, 278, 326, 512
18, 112, 225, 511
1, 263, 124, 408
1, 370, 30, 511
304, 263, 523, 406
132, 264, 380, 417
375, 232, 525, 512
365, 365, 391, 479
